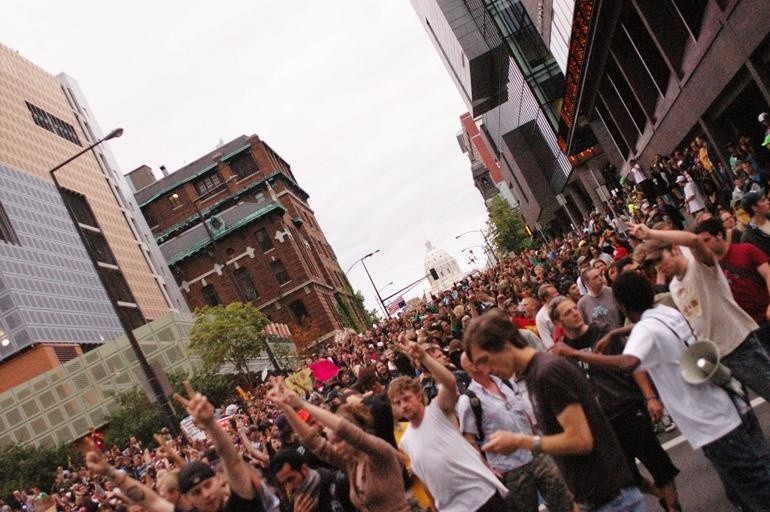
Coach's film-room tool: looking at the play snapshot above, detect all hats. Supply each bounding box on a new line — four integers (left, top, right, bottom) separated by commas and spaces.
273, 415, 291, 440
177, 459, 217, 497
742, 187, 766, 209
675, 175, 685, 183
578, 239, 586, 248
643, 237, 672, 261
324, 391, 343, 405
612, 246, 630, 261
259, 417, 273, 432
577, 255, 586, 270
730, 196, 741, 209
296, 407, 313, 422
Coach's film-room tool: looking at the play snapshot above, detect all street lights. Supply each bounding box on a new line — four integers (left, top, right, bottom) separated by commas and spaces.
359, 250, 393, 320
456, 228, 499, 268
49, 126, 191, 450
192, 174, 283, 376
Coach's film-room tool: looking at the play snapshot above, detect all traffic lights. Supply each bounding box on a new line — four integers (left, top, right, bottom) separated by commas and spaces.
430, 268, 439, 280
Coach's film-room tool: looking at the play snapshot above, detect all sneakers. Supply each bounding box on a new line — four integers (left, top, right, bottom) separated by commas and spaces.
651, 407, 676, 434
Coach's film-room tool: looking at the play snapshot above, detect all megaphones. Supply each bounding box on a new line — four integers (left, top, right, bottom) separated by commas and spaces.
679, 339, 747, 398
